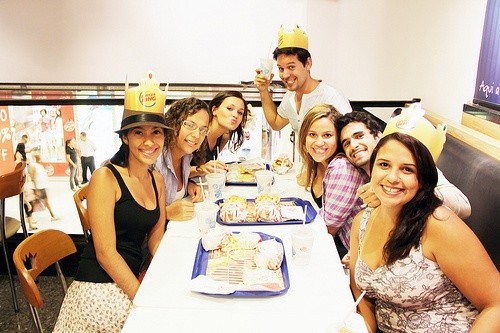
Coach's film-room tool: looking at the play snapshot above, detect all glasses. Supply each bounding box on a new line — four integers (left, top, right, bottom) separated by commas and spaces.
182, 119, 209, 136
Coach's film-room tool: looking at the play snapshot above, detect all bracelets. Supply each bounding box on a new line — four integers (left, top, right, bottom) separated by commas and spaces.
188, 180, 196, 184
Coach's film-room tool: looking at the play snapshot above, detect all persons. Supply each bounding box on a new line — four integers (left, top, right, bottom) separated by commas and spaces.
151, 98, 212, 220
255, 47, 351, 131
65, 137, 83, 191
53, 111, 166, 332
13, 136, 55, 231
340, 111, 470, 267
297, 104, 364, 259
76, 131, 95, 184
349, 132, 500, 333
189, 90, 246, 178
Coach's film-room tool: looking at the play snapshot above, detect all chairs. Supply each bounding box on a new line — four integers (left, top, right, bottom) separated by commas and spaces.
72, 184, 91, 245
12, 228, 77, 333
0, 160, 40, 314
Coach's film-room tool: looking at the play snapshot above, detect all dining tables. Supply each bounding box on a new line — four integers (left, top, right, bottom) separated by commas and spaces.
119, 158, 370, 333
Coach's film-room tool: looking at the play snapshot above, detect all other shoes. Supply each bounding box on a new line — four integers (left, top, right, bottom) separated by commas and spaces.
51, 216, 60, 221
26, 218, 37, 224
81, 179, 89, 184
29, 226, 38, 231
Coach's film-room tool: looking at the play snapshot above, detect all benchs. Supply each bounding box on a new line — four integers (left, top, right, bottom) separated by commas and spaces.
391, 103, 500, 275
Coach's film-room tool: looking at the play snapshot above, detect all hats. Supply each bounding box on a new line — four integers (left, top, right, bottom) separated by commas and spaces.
114, 109, 175, 134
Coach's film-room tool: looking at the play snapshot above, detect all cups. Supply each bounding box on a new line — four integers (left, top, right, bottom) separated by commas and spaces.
254, 170, 274, 197
205, 173, 225, 200
195, 202, 219, 232
258, 58, 275, 80
217, 164, 228, 181
292, 226, 315, 262
